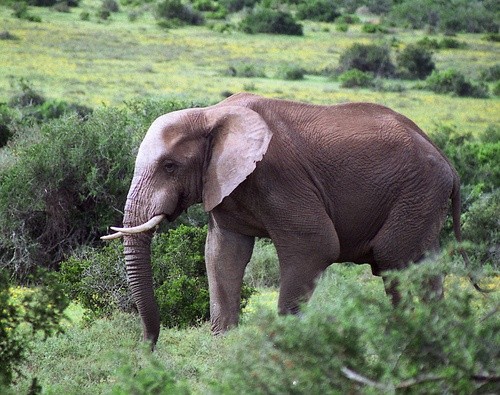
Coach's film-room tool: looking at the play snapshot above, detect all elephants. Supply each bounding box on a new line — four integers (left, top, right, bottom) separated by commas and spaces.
100, 91, 497, 357
